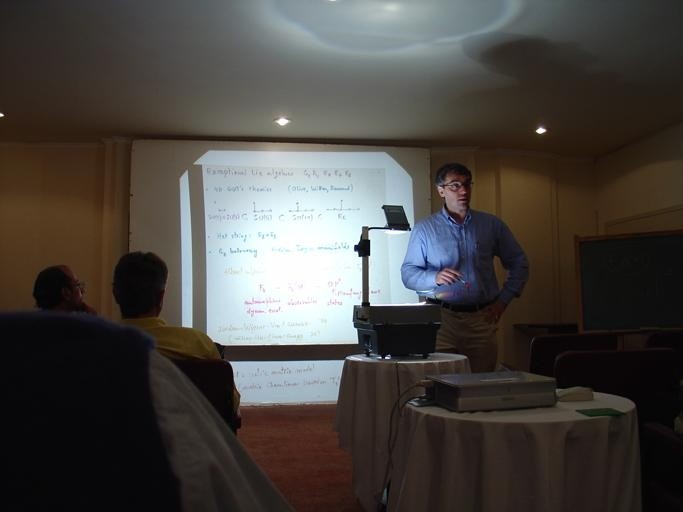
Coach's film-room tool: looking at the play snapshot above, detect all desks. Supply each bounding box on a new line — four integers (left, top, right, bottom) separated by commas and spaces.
386, 389, 643, 512
334, 350, 474, 511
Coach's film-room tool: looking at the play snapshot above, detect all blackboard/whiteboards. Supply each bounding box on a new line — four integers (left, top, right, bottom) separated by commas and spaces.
573, 233, 683, 336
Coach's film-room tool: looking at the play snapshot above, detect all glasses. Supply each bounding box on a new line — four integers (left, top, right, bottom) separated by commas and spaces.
438, 181, 475, 189
58, 280, 91, 298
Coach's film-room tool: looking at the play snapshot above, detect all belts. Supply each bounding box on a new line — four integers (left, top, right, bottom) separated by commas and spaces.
426, 295, 500, 308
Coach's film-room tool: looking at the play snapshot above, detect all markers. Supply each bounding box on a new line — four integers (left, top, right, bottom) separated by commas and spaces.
641, 327, 658, 330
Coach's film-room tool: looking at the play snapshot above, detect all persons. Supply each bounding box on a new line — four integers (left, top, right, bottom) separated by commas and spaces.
109, 251, 242, 419
30, 262, 99, 320
400, 160, 529, 373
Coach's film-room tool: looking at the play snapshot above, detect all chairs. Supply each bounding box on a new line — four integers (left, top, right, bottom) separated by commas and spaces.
524, 328, 682, 512
174, 360, 242, 436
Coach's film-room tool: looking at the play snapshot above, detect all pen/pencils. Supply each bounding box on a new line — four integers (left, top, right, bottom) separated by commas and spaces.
460, 278, 471, 288
